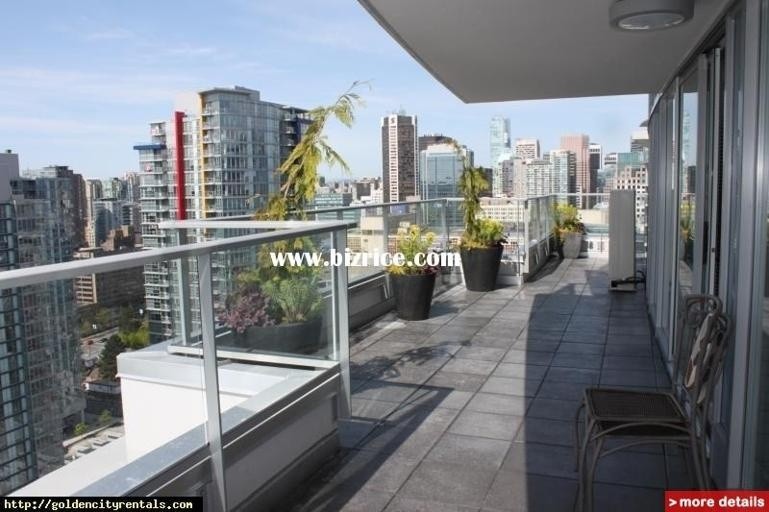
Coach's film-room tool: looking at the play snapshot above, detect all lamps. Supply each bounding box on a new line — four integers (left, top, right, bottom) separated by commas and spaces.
607, 0, 694, 32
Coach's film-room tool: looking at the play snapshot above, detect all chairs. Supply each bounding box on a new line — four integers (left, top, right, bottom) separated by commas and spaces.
574, 294, 731, 511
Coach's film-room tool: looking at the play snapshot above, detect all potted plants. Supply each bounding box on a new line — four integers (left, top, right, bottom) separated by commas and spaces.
387, 224, 439, 322
456, 166, 509, 293
679, 198, 695, 263
222, 76, 365, 368
550, 203, 586, 259
